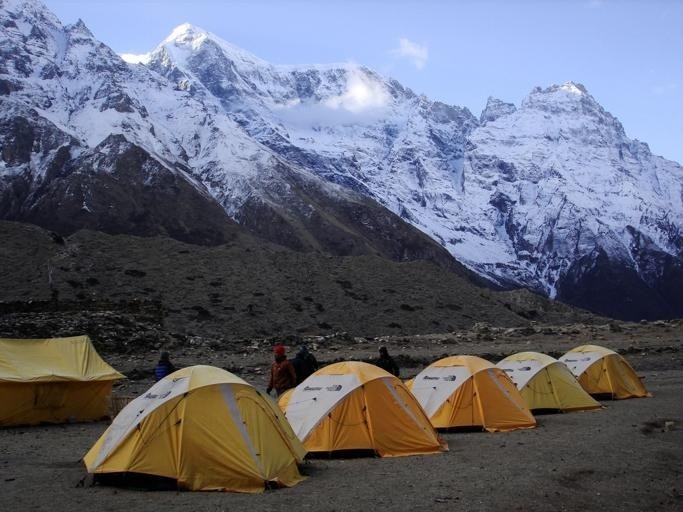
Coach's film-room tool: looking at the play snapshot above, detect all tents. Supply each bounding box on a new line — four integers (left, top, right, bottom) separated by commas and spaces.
85, 364, 308, 493
0, 334, 128, 429
559, 344, 654, 399
492, 352, 607, 413
403, 355, 536, 434
276, 361, 449, 459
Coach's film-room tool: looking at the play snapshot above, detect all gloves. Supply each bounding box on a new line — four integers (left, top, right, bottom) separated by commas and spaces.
266, 386, 272, 395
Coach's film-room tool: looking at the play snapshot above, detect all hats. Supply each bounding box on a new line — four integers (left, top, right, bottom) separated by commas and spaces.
272, 344, 285, 354
378, 346, 387, 352
159, 351, 169, 360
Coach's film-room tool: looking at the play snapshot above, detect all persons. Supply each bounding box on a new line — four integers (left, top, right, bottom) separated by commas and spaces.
364, 347, 399, 379
292, 345, 318, 385
153, 352, 182, 382
266, 346, 297, 398
